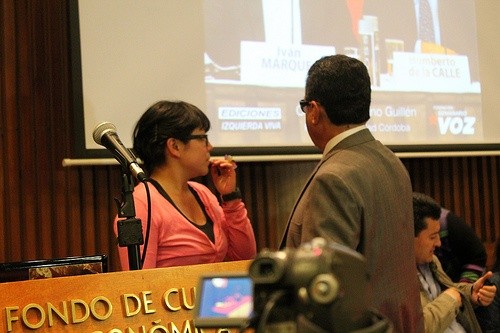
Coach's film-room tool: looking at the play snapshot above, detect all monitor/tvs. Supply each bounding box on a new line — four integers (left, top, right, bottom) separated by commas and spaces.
193, 271, 258, 327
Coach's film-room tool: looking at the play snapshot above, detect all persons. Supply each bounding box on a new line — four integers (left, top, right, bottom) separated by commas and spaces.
477, 238, 500, 333
279, 54, 425, 333
412, 191, 497, 333
114, 101, 258, 271
439, 208, 487, 283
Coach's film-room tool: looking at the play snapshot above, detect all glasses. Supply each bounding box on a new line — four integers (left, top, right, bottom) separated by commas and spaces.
188, 134, 208, 148
299, 99, 321, 113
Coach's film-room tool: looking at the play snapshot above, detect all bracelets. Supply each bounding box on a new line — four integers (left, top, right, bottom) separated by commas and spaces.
221, 188, 241, 204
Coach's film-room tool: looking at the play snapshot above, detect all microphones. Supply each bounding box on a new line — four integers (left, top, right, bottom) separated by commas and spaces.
92, 122, 148, 184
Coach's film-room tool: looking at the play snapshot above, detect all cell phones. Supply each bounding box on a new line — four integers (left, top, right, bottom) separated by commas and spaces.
477, 279, 493, 304
